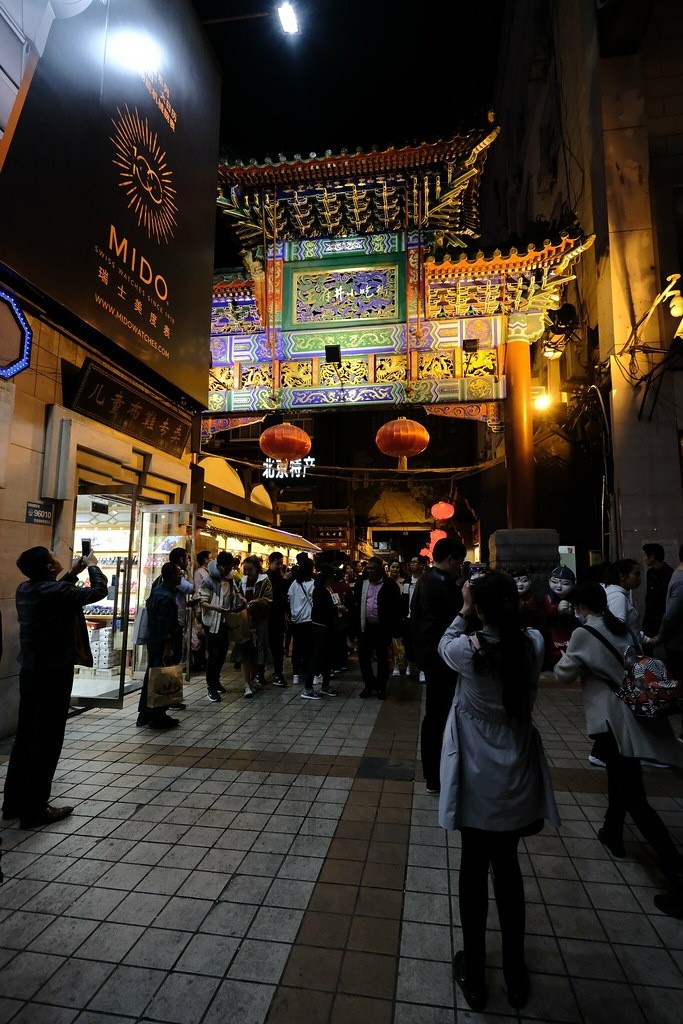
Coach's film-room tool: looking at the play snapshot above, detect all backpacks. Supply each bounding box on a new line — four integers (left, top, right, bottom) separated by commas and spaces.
582, 621, 679, 718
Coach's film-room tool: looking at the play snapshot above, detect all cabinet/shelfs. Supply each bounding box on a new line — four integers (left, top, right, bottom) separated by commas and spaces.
76, 551, 138, 594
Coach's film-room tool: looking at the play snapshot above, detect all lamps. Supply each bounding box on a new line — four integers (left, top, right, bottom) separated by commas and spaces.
325, 344, 346, 385
462, 340, 479, 378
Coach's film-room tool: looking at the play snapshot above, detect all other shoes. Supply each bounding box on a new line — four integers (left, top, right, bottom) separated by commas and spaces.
588, 746, 607, 768
426, 779, 439, 793
254, 672, 266, 686
234, 663, 241, 669
292, 663, 427, 700
2, 808, 19, 821
170, 703, 186, 708
453, 950, 489, 1011
136, 712, 150, 726
19, 803, 74, 828
251, 687, 256, 691
215, 682, 225, 694
207, 690, 220, 702
598, 828, 626, 858
243, 687, 253, 697
506, 974, 531, 1010
640, 757, 670, 768
272, 675, 287, 686
149, 715, 179, 728
654, 893, 683, 920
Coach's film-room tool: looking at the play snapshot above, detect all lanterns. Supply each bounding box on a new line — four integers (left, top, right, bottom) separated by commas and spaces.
259, 422, 311, 468
376, 419, 430, 472
431, 503, 454, 522
420, 530, 447, 562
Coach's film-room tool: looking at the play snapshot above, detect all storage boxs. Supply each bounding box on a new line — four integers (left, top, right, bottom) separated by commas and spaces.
74, 628, 123, 677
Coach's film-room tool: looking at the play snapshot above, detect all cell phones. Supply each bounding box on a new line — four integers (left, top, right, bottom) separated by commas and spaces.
82, 539, 91, 557
469, 563, 487, 586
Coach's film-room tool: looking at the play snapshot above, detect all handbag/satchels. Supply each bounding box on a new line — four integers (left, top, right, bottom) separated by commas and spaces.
388, 637, 407, 670
224, 602, 251, 644
331, 592, 343, 617
147, 658, 184, 708
136, 609, 150, 645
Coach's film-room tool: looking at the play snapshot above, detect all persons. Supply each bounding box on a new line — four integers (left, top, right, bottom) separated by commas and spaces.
642, 543, 675, 659
654, 545, 683, 743
508, 572, 535, 628
1, 546, 108, 829
404, 538, 478, 795
136, 549, 472, 729
544, 565, 575, 657
587, 560, 671, 768
438, 571, 561, 1012
552, 580, 683, 920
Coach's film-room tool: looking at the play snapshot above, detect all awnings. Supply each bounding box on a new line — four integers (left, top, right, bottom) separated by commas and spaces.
202, 510, 321, 552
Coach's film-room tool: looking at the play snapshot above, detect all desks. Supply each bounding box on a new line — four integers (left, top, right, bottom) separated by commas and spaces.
85, 615, 136, 627
